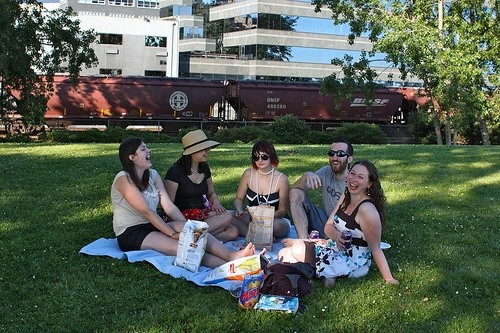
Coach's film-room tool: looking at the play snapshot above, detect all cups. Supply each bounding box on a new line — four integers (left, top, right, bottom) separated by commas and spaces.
230, 282, 242, 298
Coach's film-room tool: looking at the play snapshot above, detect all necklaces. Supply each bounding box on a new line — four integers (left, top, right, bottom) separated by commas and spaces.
256, 168, 274, 206
349, 193, 365, 208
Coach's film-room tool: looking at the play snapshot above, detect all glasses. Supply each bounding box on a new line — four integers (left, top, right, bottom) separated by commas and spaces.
328, 150, 349, 157
251, 154, 271, 160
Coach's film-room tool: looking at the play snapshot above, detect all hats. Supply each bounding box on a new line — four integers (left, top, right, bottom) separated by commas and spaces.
182, 130, 222, 156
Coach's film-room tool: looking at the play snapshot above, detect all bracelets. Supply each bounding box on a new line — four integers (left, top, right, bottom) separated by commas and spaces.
169, 232, 179, 236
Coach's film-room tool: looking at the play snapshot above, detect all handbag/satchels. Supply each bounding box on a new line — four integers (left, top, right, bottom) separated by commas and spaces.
259, 259, 315, 297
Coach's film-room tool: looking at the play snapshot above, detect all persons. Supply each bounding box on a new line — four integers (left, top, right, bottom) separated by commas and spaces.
161, 130, 238, 242
279, 159, 400, 285
111, 138, 266, 270
288, 139, 354, 239
227, 140, 291, 243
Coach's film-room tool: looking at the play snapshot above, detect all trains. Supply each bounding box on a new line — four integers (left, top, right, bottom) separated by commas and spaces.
1, 75, 458, 130
